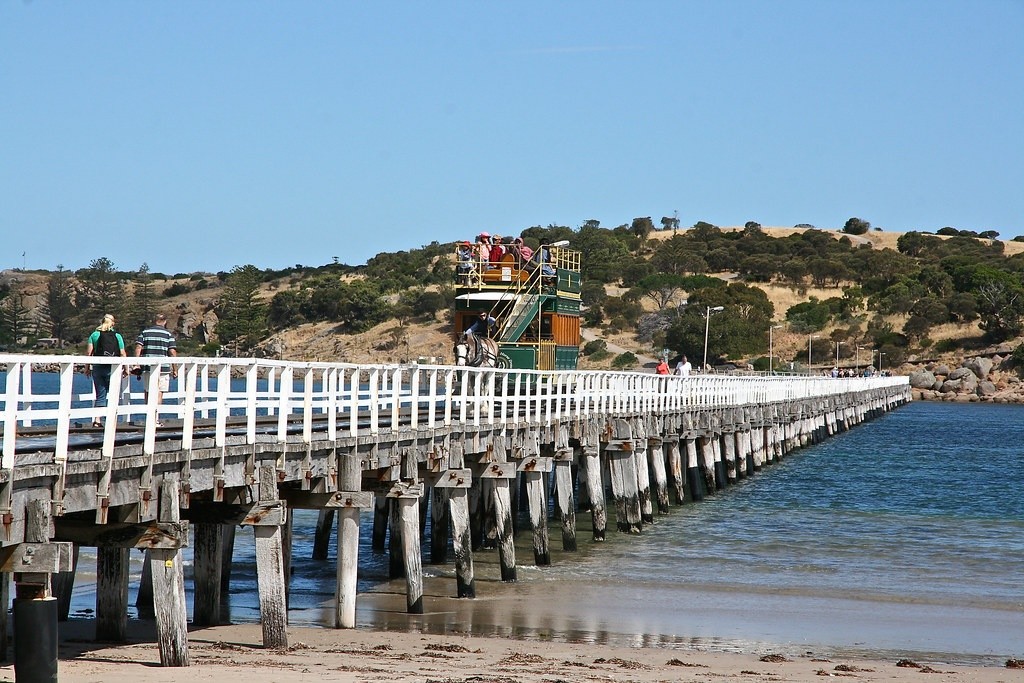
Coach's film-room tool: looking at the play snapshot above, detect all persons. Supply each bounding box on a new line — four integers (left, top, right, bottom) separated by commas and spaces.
489, 234, 509, 269
476, 234, 492, 282
746, 362, 752, 370
465, 311, 500, 343
527, 239, 555, 288
454, 240, 476, 285
824, 366, 893, 377
655, 358, 672, 408
133, 312, 178, 428
84, 314, 129, 428
509, 238, 538, 289
673, 354, 693, 404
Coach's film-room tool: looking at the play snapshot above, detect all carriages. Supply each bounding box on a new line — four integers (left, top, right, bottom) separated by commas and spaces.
454, 244, 583, 416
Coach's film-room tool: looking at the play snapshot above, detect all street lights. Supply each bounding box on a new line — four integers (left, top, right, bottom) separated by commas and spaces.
703, 306, 724, 374
857, 347, 864, 373
809, 335, 820, 376
770, 326, 782, 376
880, 352, 886, 377
872, 349, 878, 366
837, 342, 845, 371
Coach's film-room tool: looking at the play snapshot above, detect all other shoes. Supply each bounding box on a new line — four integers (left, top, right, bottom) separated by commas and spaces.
156, 422, 165, 427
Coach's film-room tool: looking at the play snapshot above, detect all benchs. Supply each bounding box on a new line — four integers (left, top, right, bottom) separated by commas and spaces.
485, 252, 530, 283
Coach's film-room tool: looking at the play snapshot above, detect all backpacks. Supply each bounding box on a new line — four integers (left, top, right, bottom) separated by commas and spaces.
94, 330, 120, 366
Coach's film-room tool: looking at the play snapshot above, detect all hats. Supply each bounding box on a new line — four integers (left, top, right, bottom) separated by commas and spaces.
658, 358, 664, 361
492, 234, 501, 240
480, 232, 491, 238
462, 241, 470, 247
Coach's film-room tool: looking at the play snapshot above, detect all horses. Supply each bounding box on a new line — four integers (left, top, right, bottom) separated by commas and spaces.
447, 329, 499, 415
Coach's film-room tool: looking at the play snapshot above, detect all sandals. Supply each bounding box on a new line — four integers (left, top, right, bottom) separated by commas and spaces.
92, 422, 103, 428
